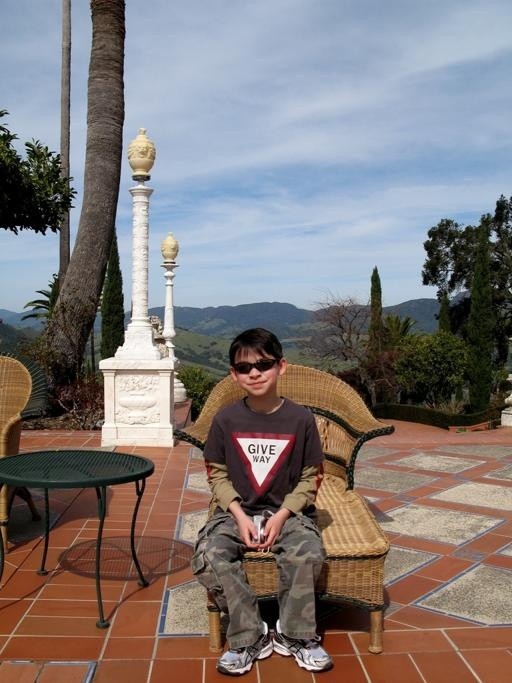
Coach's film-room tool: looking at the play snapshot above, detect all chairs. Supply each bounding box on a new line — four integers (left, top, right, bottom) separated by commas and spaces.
1, 349, 43, 557
170, 357, 396, 655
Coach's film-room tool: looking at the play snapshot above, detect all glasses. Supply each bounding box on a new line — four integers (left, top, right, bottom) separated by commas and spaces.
233, 358, 277, 374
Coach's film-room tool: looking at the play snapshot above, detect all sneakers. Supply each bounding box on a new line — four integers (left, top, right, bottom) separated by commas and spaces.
273, 617, 334, 673
216, 620, 273, 676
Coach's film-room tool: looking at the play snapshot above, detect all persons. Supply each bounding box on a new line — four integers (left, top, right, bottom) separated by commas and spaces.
190, 327, 334, 676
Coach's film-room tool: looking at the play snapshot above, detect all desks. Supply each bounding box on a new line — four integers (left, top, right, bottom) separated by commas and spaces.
2, 448, 155, 630
